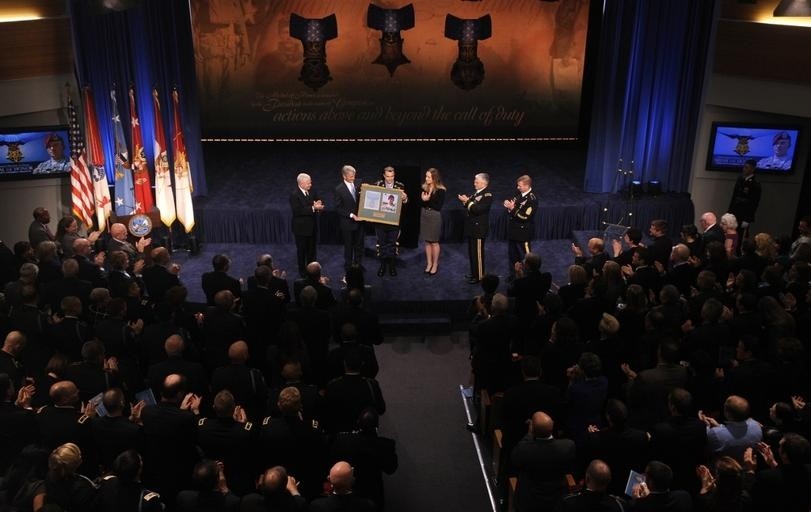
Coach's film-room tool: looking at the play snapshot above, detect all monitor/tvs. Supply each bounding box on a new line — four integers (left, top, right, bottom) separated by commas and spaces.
0, 125, 72, 182
705, 121, 802, 176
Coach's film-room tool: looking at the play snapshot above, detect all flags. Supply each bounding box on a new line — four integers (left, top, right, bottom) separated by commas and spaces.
83, 86, 113, 232
172, 90, 196, 234
153, 90, 177, 227
64, 89, 96, 230
110, 84, 136, 217
128, 90, 154, 216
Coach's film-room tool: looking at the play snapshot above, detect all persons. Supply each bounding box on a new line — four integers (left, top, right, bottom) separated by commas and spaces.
419, 168, 447, 275
335, 165, 367, 273
32, 134, 71, 175
757, 134, 792, 171
503, 175, 538, 283
290, 173, 325, 278
383, 194, 395, 212
459, 173, 496, 283
0, 208, 811, 512
728, 157, 762, 258
373, 168, 408, 277
259, 13, 306, 90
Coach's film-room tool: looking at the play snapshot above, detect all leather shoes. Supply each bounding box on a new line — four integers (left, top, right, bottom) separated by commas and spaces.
424, 269, 429, 274
429, 264, 439, 275
467, 277, 478, 284
465, 273, 472, 278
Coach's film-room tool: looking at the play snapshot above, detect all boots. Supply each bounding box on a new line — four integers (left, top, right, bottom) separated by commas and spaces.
389, 262, 396, 276
377, 263, 386, 277
